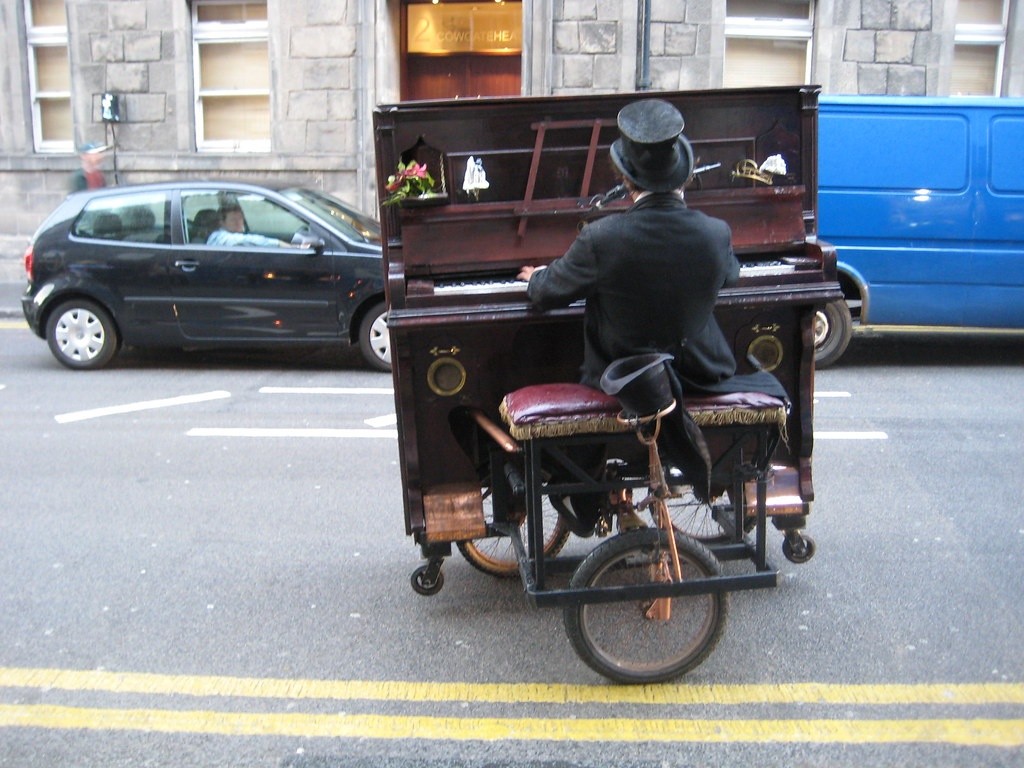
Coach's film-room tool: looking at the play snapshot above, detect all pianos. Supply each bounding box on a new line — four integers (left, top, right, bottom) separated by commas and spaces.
372, 85, 845, 597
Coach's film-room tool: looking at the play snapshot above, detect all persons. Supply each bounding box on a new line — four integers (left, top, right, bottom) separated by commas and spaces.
204, 204, 293, 249
71, 143, 108, 196
517, 99, 741, 538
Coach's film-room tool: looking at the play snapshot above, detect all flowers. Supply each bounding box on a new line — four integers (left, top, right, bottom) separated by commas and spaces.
376, 157, 435, 209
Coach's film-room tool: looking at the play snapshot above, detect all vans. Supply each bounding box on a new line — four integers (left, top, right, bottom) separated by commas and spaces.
811, 94, 1023, 370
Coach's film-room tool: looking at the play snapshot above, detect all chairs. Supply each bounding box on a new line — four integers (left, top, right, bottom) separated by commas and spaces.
92, 214, 126, 240
189, 209, 220, 243
122, 207, 165, 242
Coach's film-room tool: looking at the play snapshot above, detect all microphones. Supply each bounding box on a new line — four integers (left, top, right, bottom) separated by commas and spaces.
589, 184, 628, 214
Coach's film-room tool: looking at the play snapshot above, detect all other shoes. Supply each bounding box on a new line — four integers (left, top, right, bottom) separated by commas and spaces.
547, 476, 595, 538
665, 465, 694, 495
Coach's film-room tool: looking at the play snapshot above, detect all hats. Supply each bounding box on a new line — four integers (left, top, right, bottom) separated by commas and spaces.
609, 98, 694, 193
600, 353, 675, 419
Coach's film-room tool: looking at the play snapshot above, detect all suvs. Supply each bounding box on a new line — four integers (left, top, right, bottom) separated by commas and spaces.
20, 173, 392, 375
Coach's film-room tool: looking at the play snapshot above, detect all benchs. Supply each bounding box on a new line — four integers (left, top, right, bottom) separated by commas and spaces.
495, 370, 786, 588
